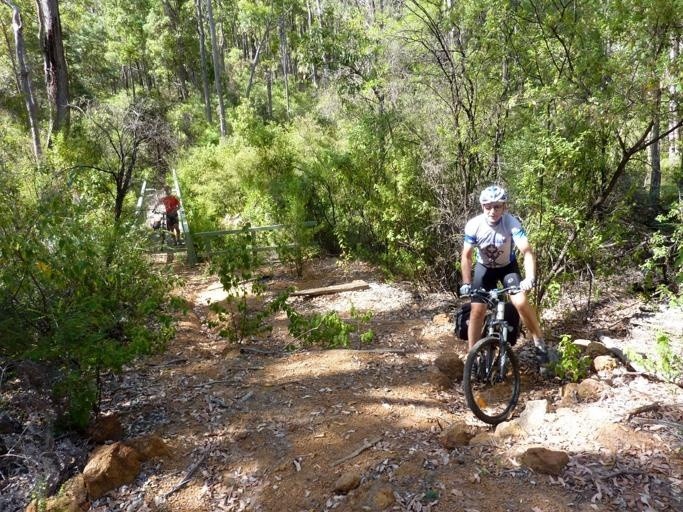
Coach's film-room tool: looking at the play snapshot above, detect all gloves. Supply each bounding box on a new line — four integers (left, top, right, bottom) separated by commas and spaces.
519, 277, 534, 291
458, 285, 470, 294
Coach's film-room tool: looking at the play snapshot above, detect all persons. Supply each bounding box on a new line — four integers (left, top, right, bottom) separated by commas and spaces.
456, 185, 549, 380
152, 185, 182, 245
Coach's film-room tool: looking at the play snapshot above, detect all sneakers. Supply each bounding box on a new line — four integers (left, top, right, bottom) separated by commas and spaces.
531, 338, 548, 355
462, 354, 478, 380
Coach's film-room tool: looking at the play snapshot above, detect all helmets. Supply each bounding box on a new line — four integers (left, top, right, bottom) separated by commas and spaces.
478, 186, 506, 204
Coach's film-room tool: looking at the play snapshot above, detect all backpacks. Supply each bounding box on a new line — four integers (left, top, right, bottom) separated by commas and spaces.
454, 302, 523, 346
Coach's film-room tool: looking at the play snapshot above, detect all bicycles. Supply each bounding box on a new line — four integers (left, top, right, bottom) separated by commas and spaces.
152, 210, 174, 250
455, 283, 537, 423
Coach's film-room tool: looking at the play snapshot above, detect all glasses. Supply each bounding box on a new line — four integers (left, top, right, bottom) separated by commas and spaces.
484, 204, 502, 209
